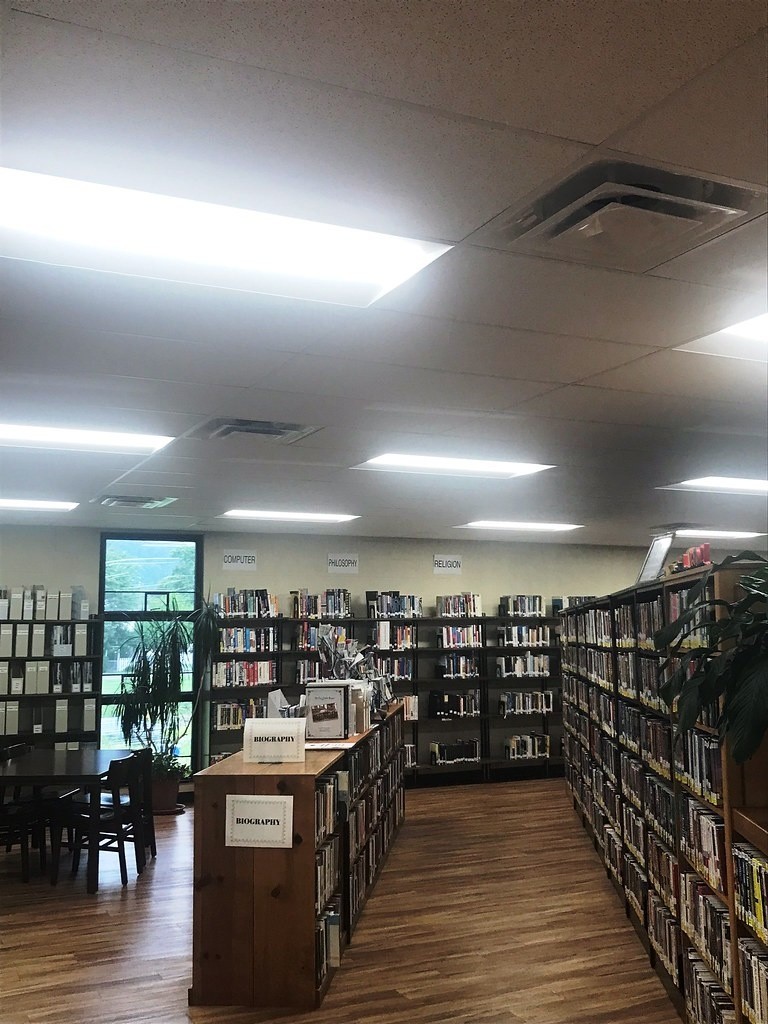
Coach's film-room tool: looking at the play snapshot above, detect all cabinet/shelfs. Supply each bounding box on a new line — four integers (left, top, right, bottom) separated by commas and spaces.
188, 560, 767, 1023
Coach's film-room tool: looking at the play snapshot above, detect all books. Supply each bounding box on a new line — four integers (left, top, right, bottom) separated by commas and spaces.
0, 583, 95, 735
209, 587, 768, 1024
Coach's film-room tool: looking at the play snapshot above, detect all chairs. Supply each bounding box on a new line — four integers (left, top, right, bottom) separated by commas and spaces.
0, 734, 81, 882
67, 746, 156, 884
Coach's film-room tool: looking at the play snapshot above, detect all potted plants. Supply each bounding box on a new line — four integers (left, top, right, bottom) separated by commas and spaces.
104, 580, 226, 815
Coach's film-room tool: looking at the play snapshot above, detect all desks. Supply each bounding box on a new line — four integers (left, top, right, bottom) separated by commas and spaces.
0, 748, 137, 893
